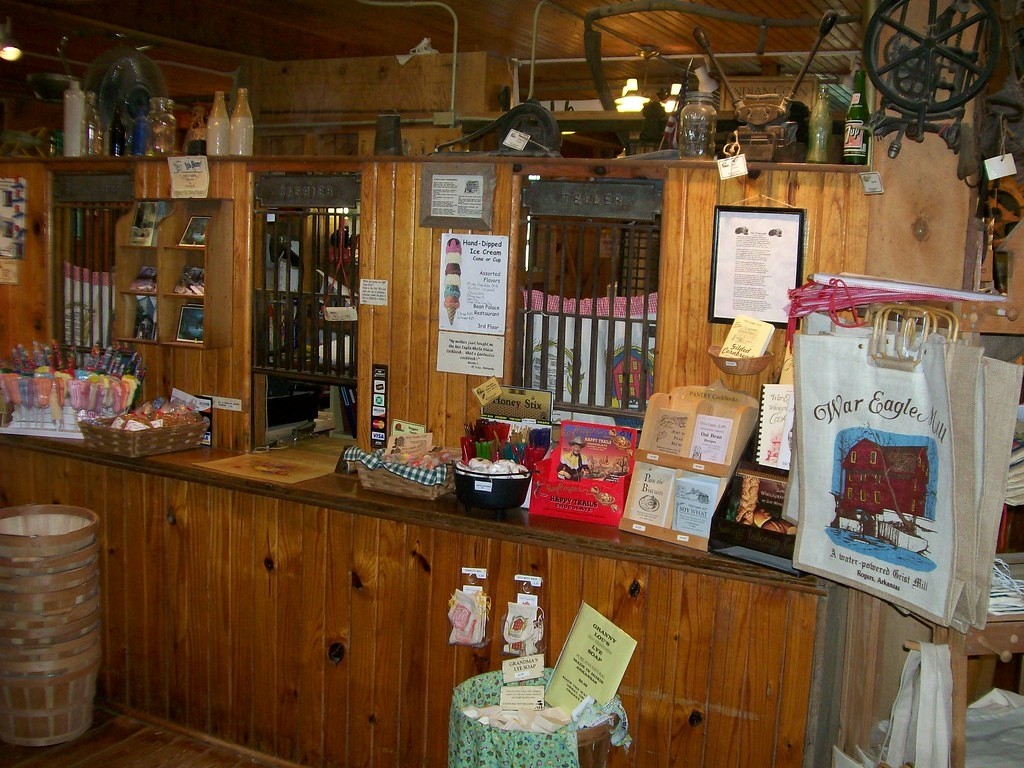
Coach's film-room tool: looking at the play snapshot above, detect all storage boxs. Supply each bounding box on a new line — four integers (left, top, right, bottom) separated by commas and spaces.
527, 419, 636, 528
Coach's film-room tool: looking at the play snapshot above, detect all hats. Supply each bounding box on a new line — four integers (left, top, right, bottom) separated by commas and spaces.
568, 437, 587, 448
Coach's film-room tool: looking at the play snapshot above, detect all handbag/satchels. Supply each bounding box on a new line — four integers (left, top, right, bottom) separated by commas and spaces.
830, 640, 1024, 767
779, 300, 1024, 637
323, 306, 358, 322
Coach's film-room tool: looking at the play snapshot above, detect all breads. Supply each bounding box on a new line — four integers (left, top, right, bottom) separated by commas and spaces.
106, 411, 198, 431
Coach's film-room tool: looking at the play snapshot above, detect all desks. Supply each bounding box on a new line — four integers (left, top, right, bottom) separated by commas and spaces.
837, 552, 1024, 768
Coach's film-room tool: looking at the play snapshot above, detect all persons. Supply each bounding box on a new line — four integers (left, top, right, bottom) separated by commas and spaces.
557, 436, 588, 480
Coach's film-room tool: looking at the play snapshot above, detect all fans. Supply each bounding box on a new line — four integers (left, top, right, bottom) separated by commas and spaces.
23, 32, 168, 137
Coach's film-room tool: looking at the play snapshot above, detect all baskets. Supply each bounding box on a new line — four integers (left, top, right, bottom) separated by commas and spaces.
77, 413, 210, 459
1, 504, 102, 748
354, 460, 456, 501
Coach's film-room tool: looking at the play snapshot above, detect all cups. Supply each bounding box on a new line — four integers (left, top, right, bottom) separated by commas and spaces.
373, 114, 403, 156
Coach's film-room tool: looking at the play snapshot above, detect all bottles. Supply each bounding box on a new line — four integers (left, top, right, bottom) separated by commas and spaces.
81, 92, 103, 156
0, 129, 44, 148
678, 91, 718, 159
843, 69, 870, 164
230, 88, 254, 156
805, 86, 830, 163
143, 97, 176, 157
206, 90, 230, 156
110, 100, 125, 156
63, 81, 86, 157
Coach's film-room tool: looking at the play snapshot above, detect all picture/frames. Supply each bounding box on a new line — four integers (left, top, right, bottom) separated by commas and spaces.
176, 303, 210, 342
179, 215, 213, 248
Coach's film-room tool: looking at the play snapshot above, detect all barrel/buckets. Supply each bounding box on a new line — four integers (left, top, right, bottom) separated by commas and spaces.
0, 503, 102, 747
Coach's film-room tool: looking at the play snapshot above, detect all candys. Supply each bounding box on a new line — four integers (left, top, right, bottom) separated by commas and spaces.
0, 340, 147, 433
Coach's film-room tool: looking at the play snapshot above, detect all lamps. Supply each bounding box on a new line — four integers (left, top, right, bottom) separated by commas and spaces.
0, 16, 15, 56
694, 57, 719, 92
610, 75, 650, 114
659, 83, 686, 115
